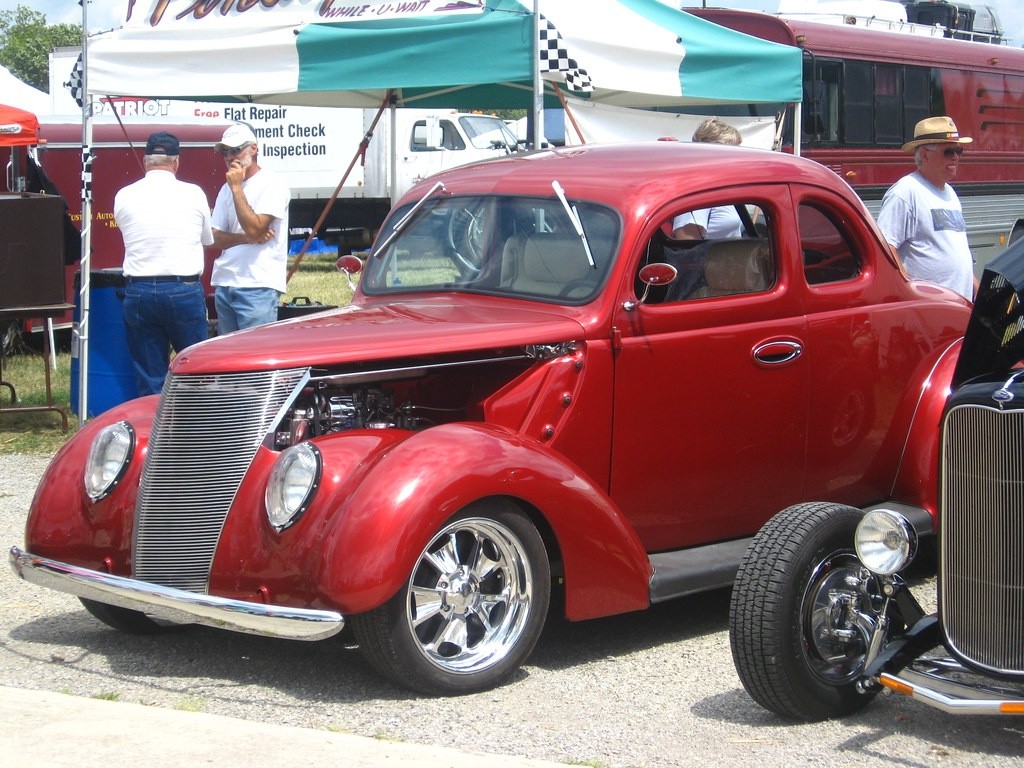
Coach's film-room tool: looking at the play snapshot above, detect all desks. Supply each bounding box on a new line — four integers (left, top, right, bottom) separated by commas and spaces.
0, 304, 77, 404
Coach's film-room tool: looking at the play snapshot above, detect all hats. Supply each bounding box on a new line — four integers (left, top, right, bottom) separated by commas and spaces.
900, 115, 978, 152
213, 123, 258, 153
143, 131, 182, 156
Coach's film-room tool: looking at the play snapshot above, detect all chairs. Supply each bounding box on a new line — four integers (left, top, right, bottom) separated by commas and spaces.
691, 240, 766, 297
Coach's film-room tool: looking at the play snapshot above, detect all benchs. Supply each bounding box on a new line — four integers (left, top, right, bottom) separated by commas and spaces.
500, 234, 613, 299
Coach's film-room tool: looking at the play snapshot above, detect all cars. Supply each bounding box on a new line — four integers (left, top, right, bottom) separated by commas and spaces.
729, 218, 1023, 728
9, 139, 979, 699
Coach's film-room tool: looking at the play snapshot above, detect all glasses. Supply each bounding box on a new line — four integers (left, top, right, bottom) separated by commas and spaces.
925, 146, 965, 159
221, 146, 249, 158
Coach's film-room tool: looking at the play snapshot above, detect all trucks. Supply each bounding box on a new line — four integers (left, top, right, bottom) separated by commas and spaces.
47, 40, 525, 257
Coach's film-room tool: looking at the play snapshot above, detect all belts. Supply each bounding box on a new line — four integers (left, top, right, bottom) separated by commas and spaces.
123, 274, 202, 283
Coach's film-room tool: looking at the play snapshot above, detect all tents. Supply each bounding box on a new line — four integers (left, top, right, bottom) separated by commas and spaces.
78, 0, 801, 426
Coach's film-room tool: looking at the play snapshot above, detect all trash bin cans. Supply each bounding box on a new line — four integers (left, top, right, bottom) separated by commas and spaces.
71, 268, 149, 422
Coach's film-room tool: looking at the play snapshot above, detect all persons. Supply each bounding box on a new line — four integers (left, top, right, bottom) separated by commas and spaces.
113, 123, 292, 394
672, 117, 743, 239
877, 117, 980, 303
